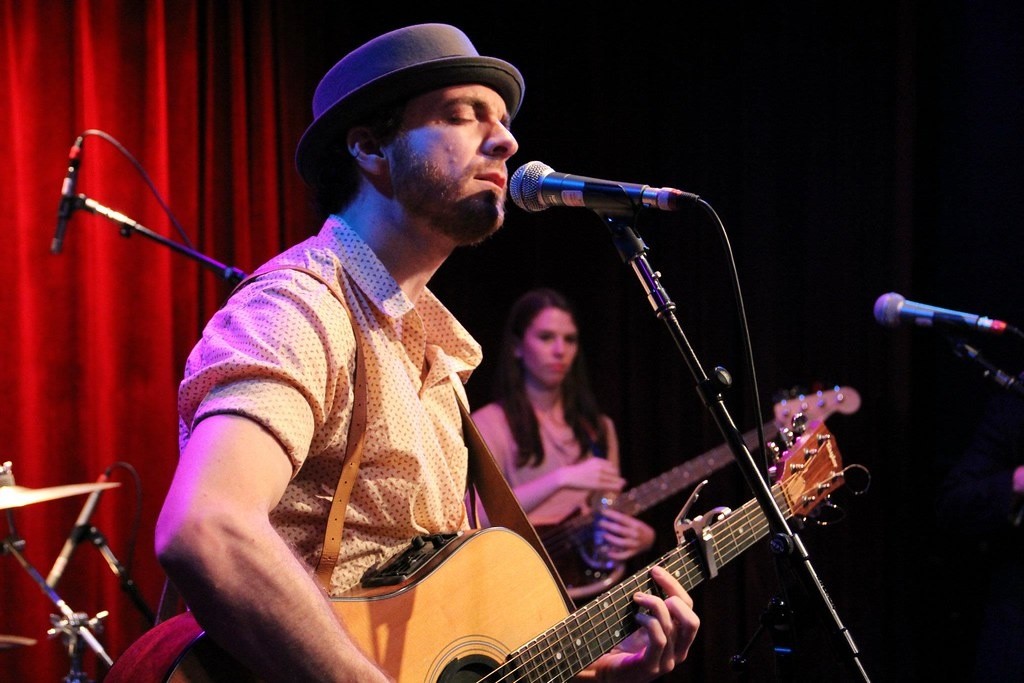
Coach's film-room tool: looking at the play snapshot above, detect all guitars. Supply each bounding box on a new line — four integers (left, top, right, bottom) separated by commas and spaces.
536, 384, 861, 599
104, 408, 844, 682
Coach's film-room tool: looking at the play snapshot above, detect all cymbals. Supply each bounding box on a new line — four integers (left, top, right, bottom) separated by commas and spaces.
0, 477, 122, 512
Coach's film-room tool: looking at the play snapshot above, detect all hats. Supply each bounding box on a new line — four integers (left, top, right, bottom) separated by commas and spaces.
296, 23, 526, 206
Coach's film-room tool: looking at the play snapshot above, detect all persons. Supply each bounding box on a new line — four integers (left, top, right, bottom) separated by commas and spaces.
151, 25, 702, 682
464, 287, 655, 611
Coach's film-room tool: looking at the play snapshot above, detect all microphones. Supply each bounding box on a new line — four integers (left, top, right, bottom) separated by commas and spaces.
46, 472, 110, 588
509, 160, 699, 212
874, 292, 1018, 334
51, 136, 84, 253
